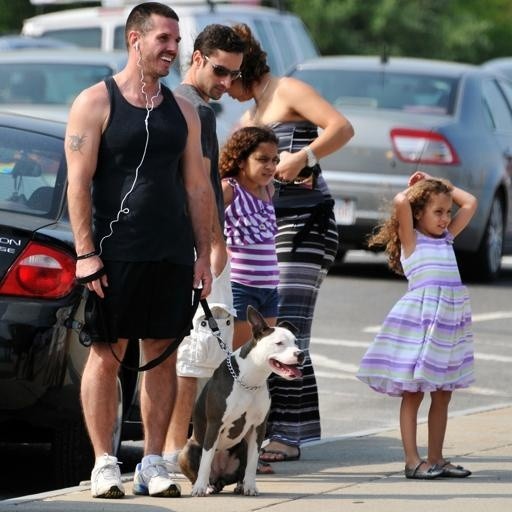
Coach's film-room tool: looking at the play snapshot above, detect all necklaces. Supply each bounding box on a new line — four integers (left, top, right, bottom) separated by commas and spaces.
255, 77, 272, 104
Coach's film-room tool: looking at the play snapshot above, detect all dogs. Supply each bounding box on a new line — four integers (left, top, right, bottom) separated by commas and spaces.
175, 304, 307, 498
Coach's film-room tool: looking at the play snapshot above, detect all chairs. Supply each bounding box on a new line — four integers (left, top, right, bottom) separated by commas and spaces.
26, 186, 56, 211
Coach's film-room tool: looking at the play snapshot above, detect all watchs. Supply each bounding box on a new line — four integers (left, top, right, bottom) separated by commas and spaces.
302, 145, 320, 171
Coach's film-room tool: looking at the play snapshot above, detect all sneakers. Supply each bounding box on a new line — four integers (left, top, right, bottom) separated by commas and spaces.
132, 454, 181, 498
90, 454, 125, 498
161, 450, 188, 479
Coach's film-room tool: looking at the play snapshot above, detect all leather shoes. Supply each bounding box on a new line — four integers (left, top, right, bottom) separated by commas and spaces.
405, 458, 472, 479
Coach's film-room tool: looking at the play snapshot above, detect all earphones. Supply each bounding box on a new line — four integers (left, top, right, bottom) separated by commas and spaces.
134, 40, 140, 51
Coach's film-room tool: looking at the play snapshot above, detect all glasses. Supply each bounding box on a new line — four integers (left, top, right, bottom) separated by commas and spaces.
204, 55, 242, 81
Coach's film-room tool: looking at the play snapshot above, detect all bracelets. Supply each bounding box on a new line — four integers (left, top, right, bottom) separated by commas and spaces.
73, 248, 98, 260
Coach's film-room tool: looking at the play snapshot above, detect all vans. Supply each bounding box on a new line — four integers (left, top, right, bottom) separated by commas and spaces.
22, 1, 325, 165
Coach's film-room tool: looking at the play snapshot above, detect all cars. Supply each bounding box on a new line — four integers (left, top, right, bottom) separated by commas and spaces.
480, 56, 511, 82
274, 40, 511, 287
1, 35, 77, 49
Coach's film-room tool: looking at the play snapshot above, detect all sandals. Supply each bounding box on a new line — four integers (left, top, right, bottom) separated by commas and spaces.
257, 433, 301, 474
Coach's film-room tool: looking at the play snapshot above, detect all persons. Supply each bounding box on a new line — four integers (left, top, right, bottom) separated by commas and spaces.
64, 3, 210, 497
222, 127, 283, 476
357, 171, 482, 479
223, 21, 356, 463
169, 24, 244, 490
